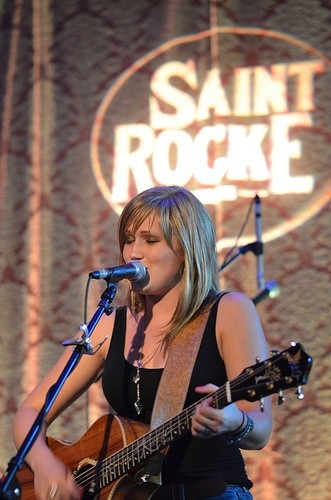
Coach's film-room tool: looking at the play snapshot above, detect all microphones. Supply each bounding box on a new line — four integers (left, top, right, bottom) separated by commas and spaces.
88, 260, 147, 283
253, 282, 280, 305
255, 196, 265, 289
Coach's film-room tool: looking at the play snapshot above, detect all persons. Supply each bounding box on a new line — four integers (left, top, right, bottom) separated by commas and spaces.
12, 185, 274, 500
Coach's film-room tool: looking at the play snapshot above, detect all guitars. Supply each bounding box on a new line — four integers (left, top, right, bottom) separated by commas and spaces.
0, 337, 314, 500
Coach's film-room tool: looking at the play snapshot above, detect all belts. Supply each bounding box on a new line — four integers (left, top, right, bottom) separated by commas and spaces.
154, 478, 227, 500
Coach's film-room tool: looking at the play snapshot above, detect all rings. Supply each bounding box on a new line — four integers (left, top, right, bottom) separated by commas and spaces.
46, 483, 57, 499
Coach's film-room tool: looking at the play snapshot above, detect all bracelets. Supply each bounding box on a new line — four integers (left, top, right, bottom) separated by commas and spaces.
223, 411, 254, 443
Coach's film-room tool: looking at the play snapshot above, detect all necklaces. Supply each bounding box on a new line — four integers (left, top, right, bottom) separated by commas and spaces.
134, 311, 162, 415
